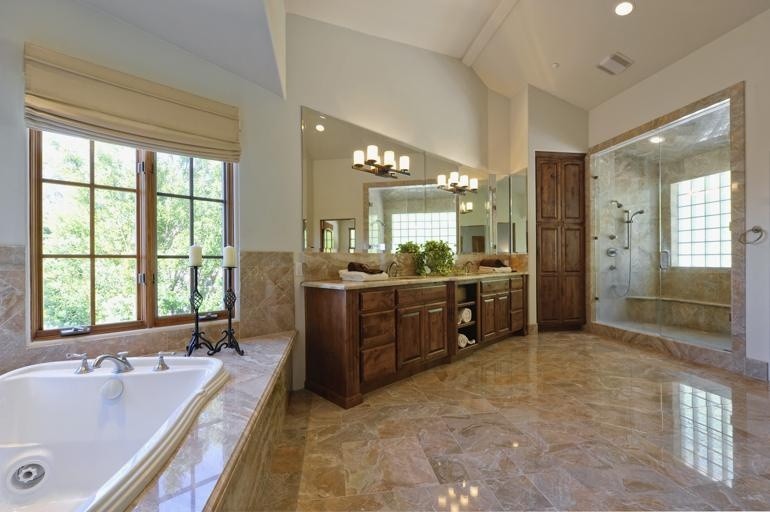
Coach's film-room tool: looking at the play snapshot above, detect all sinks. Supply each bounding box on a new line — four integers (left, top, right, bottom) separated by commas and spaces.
458, 272, 488, 275
387, 276, 430, 280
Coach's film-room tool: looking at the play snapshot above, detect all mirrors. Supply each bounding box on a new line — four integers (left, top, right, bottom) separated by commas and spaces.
301, 104, 528, 253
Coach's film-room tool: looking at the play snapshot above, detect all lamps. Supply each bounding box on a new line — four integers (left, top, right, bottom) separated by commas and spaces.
351, 151, 399, 179
437, 175, 466, 196
366, 145, 411, 176
460, 201, 473, 215
450, 172, 478, 193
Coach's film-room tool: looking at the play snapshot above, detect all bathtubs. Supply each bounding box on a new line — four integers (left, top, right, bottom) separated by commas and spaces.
0, 355, 229, 512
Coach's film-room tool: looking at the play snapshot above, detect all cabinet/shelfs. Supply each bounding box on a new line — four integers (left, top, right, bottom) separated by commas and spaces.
300, 273, 530, 410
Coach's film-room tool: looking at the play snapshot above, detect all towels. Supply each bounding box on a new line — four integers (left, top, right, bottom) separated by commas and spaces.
456, 308, 472, 324
457, 329, 476, 348
478, 258, 513, 273
338, 261, 389, 282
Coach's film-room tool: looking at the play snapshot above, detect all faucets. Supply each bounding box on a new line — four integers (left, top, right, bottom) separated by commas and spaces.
92, 351, 134, 372
462, 261, 474, 270
387, 261, 399, 276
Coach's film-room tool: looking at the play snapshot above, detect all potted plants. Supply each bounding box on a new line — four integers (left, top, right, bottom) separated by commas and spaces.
414, 240, 455, 276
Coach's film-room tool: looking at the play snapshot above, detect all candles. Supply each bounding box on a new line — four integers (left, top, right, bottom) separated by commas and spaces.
223, 246, 236, 267
189, 245, 202, 266
